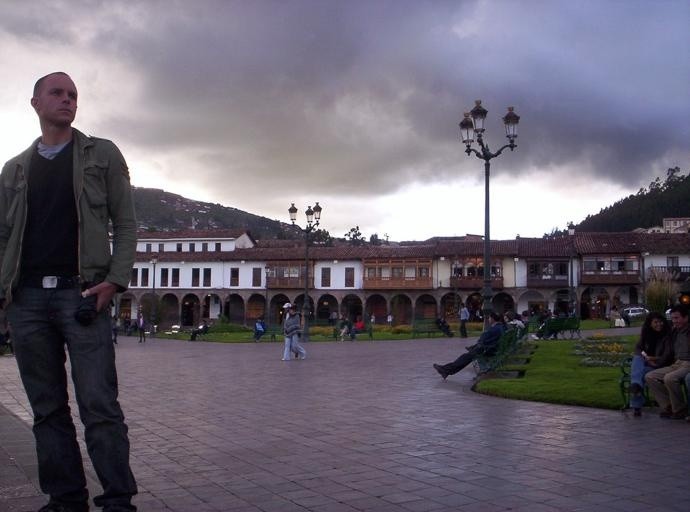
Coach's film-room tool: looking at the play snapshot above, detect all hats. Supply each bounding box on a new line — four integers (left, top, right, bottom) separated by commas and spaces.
502, 310, 514, 321
282, 302, 292, 309
488, 311, 501, 322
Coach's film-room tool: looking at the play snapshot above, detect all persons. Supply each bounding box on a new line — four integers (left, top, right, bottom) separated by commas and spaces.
111, 311, 149, 344
252, 317, 266, 342
1, 71, 137, 509
628, 312, 669, 417
458, 302, 470, 338
188, 319, 209, 342
608, 305, 631, 328
433, 308, 528, 381
280, 303, 306, 360
646, 305, 690, 418
528, 308, 569, 340
283, 302, 302, 359
387, 310, 393, 327
328, 311, 375, 343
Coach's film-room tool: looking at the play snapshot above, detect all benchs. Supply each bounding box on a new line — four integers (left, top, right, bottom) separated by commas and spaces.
607, 314, 637, 329
199, 325, 217, 342
333, 322, 373, 341
252, 324, 283, 341
472, 315, 581, 380
618, 356, 690, 412
410, 320, 447, 338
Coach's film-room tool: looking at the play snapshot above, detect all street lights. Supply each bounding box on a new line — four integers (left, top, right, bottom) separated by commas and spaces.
285, 200, 322, 342
150, 251, 159, 339
456, 98, 524, 339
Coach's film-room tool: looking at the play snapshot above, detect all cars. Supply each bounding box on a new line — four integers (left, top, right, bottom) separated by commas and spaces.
604, 303, 673, 322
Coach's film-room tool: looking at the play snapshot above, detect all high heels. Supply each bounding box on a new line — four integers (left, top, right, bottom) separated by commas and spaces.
622, 382, 643, 397
632, 408, 642, 417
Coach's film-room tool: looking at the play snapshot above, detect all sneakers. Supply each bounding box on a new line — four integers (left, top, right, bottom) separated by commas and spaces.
295, 352, 299, 359
281, 356, 291, 361
300, 349, 306, 360
659, 407, 690, 422
432, 362, 450, 380
37, 495, 90, 512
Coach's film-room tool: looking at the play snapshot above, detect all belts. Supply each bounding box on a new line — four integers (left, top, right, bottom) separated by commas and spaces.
18, 272, 82, 291
